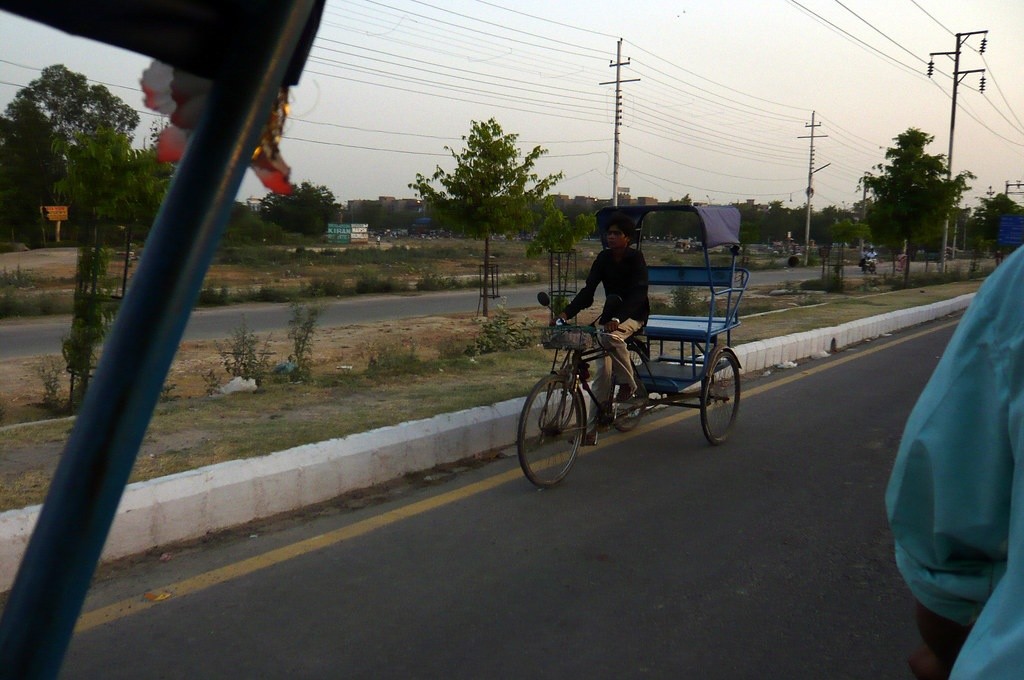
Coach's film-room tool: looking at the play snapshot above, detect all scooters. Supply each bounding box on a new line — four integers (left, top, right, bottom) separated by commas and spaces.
860, 254, 878, 274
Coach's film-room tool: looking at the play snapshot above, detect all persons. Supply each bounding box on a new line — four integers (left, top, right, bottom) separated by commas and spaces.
887, 248, 1024, 680
551, 215, 650, 443
898, 249, 907, 271
861, 247, 877, 273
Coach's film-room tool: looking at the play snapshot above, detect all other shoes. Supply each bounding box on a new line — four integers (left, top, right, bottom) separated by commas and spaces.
615, 383, 634, 402
568, 433, 596, 446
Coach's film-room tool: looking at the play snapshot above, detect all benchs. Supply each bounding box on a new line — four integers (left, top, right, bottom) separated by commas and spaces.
635, 319, 726, 341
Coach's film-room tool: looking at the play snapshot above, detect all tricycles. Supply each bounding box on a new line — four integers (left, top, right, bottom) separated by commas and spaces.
515, 203, 749, 489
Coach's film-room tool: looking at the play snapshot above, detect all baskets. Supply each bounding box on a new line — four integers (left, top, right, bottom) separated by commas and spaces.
541, 326, 595, 350
